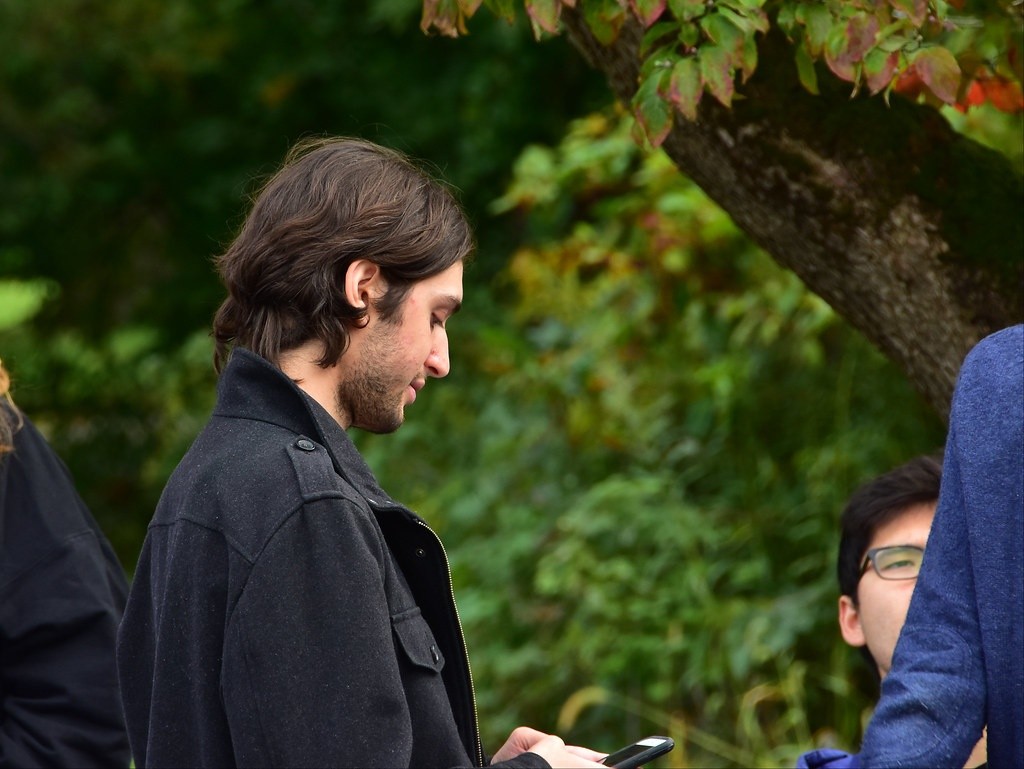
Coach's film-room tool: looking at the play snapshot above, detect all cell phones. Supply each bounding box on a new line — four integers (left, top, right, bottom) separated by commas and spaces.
597, 735, 675, 769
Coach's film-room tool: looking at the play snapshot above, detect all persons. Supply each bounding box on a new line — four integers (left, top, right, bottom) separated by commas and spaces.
1, 369, 131, 769
847, 323, 1024, 769
115, 136, 609, 769
797, 445, 987, 769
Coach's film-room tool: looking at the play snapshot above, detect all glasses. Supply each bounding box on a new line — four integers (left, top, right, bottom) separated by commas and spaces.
856, 544, 926, 580
